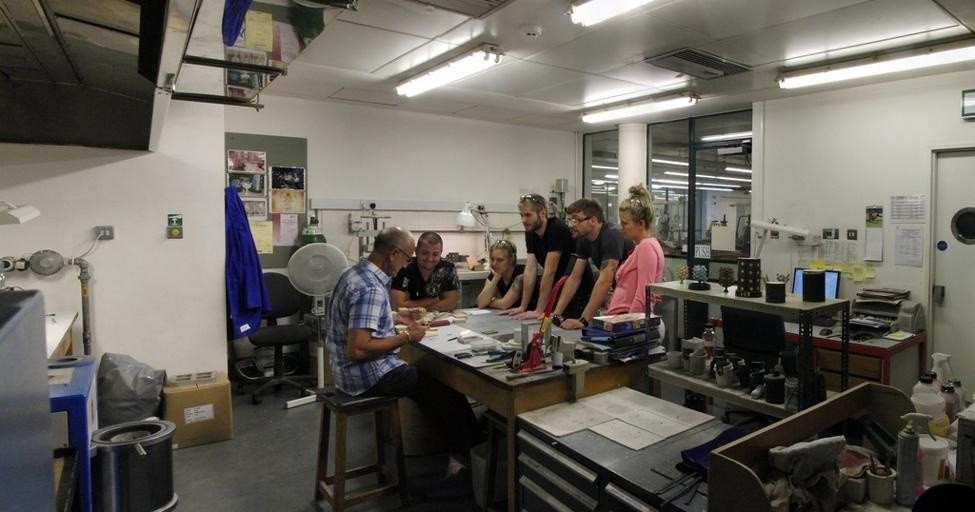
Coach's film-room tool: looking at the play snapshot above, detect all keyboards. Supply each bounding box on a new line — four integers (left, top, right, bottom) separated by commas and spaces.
789, 318, 837, 327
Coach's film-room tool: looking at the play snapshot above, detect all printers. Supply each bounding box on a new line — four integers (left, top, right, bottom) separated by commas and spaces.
852, 294, 926, 333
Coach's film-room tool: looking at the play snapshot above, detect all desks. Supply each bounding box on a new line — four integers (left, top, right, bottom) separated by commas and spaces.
393, 305, 672, 512
450, 258, 546, 309
45, 351, 105, 512
703, 317, 929, 400
38, 309, 83, 362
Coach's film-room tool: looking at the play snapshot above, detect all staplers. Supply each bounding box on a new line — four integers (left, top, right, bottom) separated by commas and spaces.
487, 351, 508, 364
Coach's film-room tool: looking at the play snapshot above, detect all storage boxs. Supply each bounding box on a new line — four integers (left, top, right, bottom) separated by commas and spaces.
950, 406, 975, 488
159, 369, 233, 452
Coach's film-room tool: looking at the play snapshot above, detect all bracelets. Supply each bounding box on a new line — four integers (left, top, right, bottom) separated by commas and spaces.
401, 330, 413, 343
579, 317, 589, 328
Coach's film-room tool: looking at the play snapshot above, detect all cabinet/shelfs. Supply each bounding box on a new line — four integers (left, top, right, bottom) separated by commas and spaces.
1, 281, 59, 511
54, 442, 84, 512
638, 276, 853, 429
509, 388, 751, 512
701, 376, 929, 511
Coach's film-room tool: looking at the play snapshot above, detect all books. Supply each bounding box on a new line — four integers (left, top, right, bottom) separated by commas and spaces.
856, 286, 911, 300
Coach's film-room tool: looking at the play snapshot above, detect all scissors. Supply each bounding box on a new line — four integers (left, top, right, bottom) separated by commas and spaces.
455, 352, 487, 359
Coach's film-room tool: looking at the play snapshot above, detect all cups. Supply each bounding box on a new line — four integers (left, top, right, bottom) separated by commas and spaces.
513, 328, 522, 342
552, 351, 563, 369
765, 281, 785, 303
803, 270, 825, 302
867, 466, 896, 504
666, 350, 800, 412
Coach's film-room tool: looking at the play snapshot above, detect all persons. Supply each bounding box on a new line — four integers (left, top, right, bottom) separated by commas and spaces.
549, 199, 636, 331
603, 184, 666, 315
324, 225, 489, 449
388, 229, 460, 314
477, 238, 540, 309
496, 193, 595, 318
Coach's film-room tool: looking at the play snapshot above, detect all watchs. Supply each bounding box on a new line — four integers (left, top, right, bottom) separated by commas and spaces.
489, 296, 496, 304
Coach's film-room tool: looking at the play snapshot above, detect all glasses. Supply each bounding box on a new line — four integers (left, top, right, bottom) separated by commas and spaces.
567, 216, 591, 225
519, 194, 544, 206
496, 239, 516, 252
394, 244, 414, 264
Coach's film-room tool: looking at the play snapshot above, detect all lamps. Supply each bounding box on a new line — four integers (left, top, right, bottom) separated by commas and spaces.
750, 218, 811, 261
774, 28, 974, 96
390, 40, 510, 109
1, 198, 42, 228
454, 197, 497, 271
297, 214, 328, 245
568, 0, 654, 33
579, 86, 706, 128
546, 178, 571, 222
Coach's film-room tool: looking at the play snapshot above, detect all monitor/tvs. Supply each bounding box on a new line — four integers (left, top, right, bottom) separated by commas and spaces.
793, 264, 841, 300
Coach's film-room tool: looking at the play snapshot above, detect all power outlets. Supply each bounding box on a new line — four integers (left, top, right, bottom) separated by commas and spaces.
93, 225, 116, 244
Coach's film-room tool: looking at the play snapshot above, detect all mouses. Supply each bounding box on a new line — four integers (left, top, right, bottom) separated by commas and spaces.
820, 330, 833, 336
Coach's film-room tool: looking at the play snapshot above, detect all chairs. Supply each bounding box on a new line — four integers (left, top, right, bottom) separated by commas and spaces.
241, 271, 322, 410
718, 302, 800, 426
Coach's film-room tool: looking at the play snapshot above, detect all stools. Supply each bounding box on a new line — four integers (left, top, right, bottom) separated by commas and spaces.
307, 383, 412, 511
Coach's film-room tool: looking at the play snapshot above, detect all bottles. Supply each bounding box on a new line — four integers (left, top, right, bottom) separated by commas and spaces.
911, 369, 967, 428
703, 322, 716, 357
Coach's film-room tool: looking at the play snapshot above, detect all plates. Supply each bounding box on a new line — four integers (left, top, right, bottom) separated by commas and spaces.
508, 340, 521, 346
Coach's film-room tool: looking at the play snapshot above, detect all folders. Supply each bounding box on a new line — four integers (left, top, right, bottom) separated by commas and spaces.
581, 327, 661, 349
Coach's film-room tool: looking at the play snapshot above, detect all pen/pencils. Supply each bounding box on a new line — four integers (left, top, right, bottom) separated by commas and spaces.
763, 274, 769, 283
886, 452, 893, 474
448, 337, 457, 341
870, 453, 877, 475
826, 335, 841, 338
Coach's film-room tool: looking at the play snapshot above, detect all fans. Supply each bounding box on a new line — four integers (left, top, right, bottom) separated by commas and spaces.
285, 244, 350, 410
